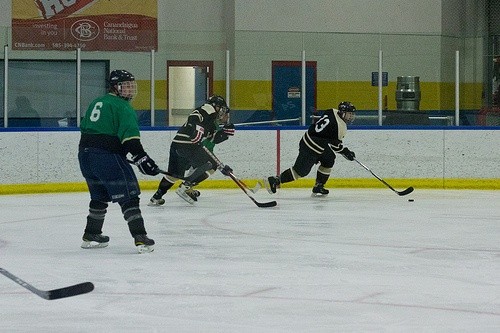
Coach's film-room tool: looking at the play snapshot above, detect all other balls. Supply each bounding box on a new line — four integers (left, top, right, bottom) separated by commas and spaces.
408, 199, 414, 202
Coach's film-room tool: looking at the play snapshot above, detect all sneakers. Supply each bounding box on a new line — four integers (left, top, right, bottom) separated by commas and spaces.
147, 195, 165, 207
265, 175, 280, 194
135, 234, 156, 254
81, 233, 110, 248
176, 183, 200, 205
310, 185, 330, 199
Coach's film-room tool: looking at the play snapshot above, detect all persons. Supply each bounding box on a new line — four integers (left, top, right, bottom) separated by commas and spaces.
263, 101, 356, 196
148, 96, 236, 208
78, 69, 161, 255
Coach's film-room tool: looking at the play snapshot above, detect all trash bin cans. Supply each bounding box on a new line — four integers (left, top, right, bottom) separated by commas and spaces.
395, 76, 421, 110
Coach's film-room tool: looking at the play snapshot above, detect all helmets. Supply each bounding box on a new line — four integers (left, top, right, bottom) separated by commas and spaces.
208, 95, 227, 116
105, 69, 138, 102
337, 101, 357, 124
217, 106, 230, 125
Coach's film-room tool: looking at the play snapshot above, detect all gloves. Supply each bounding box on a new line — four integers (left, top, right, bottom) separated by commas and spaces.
340, 147, 355, 161
190, 130, 215, 150
222, 122, 235, 136
131, 150, 160, 176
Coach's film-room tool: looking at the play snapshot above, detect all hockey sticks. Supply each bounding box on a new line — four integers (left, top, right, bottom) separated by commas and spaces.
0, 267, 95, 301
126, 160, 216, 186
353, 156, 415, 196
201, 145, 278, 208
